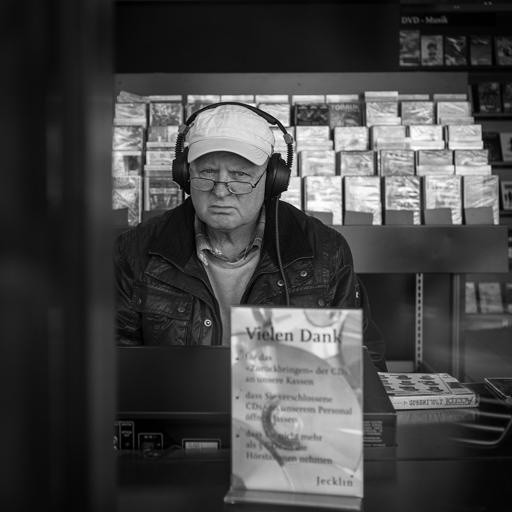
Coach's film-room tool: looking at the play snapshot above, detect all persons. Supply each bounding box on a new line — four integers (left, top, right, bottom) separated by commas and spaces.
114, 103, 362, 344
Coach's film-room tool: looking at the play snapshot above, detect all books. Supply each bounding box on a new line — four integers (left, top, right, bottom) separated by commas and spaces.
114, 90, 502, 227
379, 371, 477, 410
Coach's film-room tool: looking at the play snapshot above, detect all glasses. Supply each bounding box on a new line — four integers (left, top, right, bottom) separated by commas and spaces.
187, 169, 268, 196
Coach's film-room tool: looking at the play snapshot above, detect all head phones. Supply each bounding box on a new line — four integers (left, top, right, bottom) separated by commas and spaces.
170, 102, 295, 202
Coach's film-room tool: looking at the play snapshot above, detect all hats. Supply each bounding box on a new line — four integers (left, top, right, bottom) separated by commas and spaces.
185, 103, 277, 168
